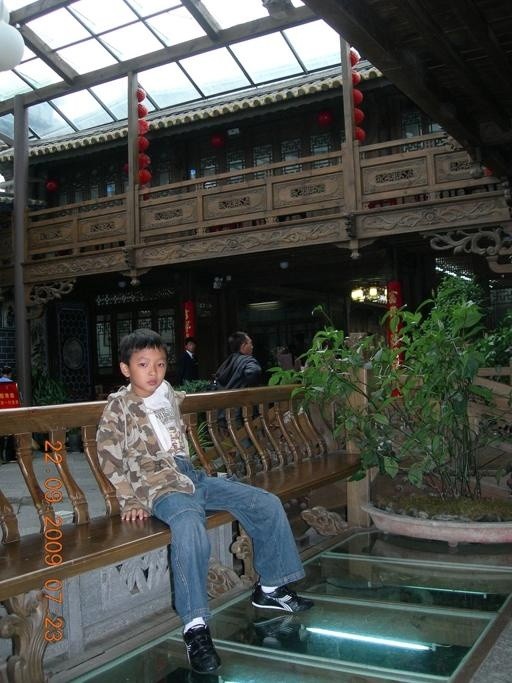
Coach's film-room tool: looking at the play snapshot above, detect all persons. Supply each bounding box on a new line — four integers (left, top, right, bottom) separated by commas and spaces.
95, 326, 314, 676
175, 336, 199, 384
214, 332, 262, 461
1, 365, 20, 464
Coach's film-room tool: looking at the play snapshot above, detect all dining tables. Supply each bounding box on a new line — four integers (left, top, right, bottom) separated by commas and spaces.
258, 260, 510, 549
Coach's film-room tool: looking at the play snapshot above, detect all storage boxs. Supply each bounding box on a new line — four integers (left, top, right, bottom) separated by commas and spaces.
1, 381, 381, 681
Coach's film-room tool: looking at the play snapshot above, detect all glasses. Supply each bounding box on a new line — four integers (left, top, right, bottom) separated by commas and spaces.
343, 272, 394, 306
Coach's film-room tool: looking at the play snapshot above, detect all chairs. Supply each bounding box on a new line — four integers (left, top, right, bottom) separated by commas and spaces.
251, 583, 315, 613
182, 622, 223, 674
